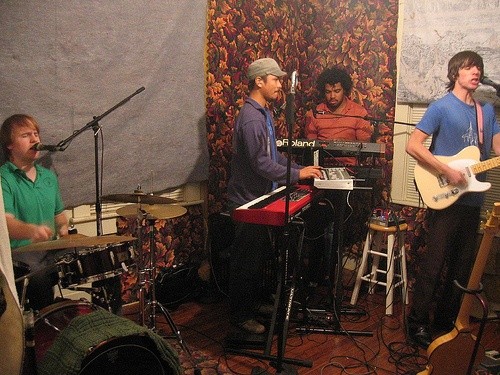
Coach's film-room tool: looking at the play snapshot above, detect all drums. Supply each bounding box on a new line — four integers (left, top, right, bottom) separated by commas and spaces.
74, 239, 147, 282
25, 298, 177, 375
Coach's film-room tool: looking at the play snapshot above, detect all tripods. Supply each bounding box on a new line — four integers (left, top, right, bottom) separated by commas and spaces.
133, 219, 203, 375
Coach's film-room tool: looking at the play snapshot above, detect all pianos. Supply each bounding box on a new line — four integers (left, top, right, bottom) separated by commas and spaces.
313, 159, 358, 191
221, 170, 339, 369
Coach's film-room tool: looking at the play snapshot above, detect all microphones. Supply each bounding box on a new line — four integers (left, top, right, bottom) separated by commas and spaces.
293, 70, 299, 93
310, 103, 317, 118
32, 142, 65, 151
479, 75, 500, 96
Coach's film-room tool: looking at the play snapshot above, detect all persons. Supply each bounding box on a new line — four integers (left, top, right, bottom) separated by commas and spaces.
405, 50, 500, 348
302, 66, 374, 281
226, 57, 325, 334
0, 113, 69, 311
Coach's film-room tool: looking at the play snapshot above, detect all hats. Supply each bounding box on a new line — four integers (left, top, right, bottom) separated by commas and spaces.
248, 58, 287, 80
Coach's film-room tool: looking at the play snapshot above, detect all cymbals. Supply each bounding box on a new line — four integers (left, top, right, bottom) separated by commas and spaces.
12, 232, 141, 253
114, 204, 188, 222
96, 193, 179, 205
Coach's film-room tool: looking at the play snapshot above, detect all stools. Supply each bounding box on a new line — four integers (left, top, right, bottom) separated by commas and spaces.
350, 219, 410, 317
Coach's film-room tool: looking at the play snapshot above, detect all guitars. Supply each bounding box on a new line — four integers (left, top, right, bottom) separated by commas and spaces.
415, 201, 500, 374
407, 145, 500, 211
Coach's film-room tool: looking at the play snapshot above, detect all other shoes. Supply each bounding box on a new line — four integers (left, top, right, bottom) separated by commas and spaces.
406, 322, 433, 347
236, 318, 265, 333
251, 302, 273, 313
433, 316, 456, 330
